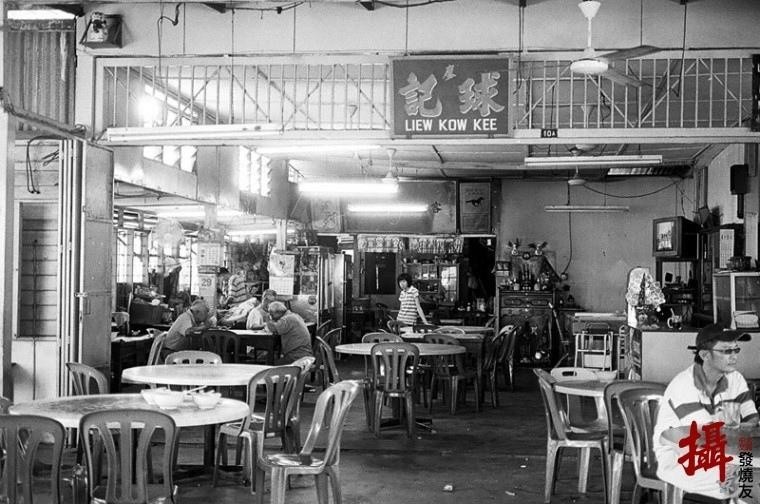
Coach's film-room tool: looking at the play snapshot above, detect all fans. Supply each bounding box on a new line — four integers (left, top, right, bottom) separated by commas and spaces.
381, 147, 447, 185
155, 217, 186, 249
503, 1, 663, 88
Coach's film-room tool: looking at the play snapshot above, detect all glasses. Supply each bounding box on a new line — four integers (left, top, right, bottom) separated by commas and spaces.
710, 347, 742, 354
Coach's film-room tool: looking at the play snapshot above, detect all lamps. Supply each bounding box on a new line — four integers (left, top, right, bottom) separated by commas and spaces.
523, 154, 664, 171
543, 204, 630, 213
106, 123, 288, 145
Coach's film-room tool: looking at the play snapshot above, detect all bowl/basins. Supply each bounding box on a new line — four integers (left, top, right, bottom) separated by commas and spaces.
596, 371, 616, 383
141, 389, 162, 405
192, 393, 221, 409
152, 391, 183, 410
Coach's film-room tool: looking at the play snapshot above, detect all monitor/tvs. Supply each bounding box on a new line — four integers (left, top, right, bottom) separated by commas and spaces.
652, 216, 696, 261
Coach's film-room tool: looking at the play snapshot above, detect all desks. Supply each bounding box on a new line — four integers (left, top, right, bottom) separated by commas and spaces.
623, 322, 759, 383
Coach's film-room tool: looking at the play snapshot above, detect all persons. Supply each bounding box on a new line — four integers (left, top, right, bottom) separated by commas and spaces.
216, 266, 247, 309
265, 300, 314, 365
160, 304, 214, 360
245, 288, 277, 359
651, 322, 760, 504
396, 273, 429, 327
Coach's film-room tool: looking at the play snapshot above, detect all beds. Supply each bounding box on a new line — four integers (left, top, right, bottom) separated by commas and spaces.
661, 422, 759, 464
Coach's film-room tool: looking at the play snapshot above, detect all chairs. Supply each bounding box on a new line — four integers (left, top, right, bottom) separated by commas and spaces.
361, 330, 405, 377
604, 382, 667, 504
66, 361, 109, 396
550, 368, 631, 494
616, 387, 683, 504
147, 326, 169, 352
255, 379, 361, 504
250, 355, 317, 450
498, 324, 518, 393
315, 335, 371, 430
201, 328, 239, 364
209, 364, 302, 496
475, 329, 508, 409
532, 366, 611, 503
165, 349, 224, 400
432, 326, 467, 332
0, 413, 65, 504
386, 320, 407, 335
412, 323, 438, 333
422, 333, 481, 416
77, 408, 182, 504
371, 342, 421, 441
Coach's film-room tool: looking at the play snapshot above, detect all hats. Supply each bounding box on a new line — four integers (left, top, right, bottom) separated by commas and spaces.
687, 324, 752, 350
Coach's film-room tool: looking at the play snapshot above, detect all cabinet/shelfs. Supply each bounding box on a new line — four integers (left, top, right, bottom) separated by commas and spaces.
495, 286, 557, 368
275, 247, 329, 311
405, 264, 459, 304
712, 271, 760, 329
